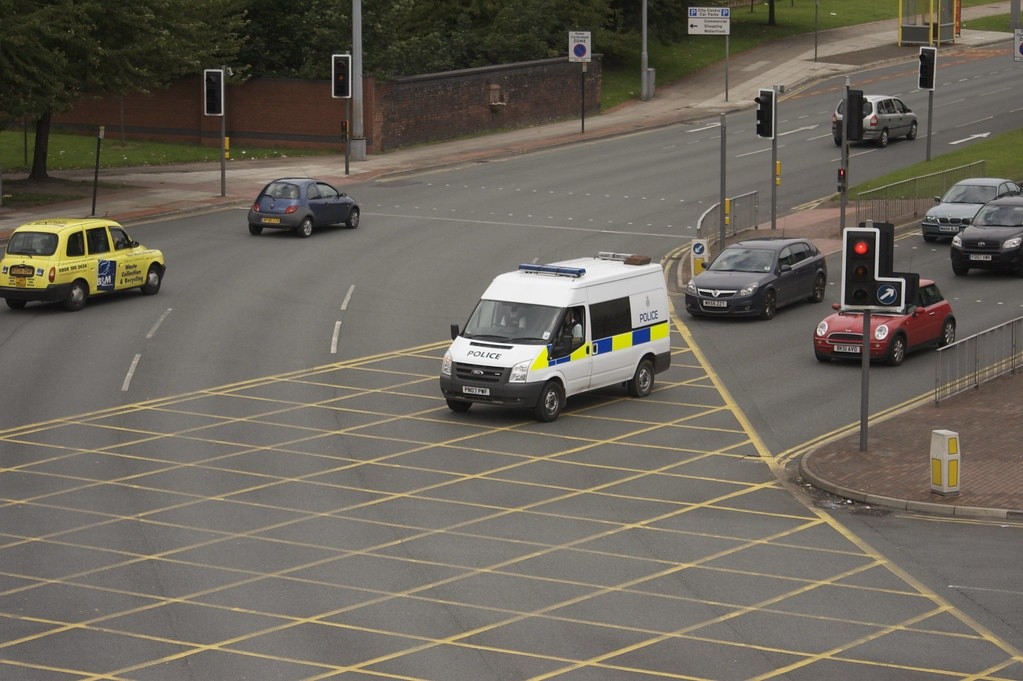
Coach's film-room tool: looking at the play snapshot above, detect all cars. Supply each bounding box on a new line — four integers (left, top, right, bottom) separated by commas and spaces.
921, 177, 1023, 242
685, 236, 827, 320
813, 279, 957, 366
247, 176, 360, 237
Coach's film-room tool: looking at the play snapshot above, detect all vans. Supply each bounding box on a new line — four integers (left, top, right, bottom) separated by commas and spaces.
440, 251, 671, 421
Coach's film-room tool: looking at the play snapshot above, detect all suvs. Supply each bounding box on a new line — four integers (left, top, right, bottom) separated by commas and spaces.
0, 216, 167, 312
950, 191, 1023, 275
832, 96, 918, 147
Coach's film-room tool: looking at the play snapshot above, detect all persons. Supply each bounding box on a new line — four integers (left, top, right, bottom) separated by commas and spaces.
500, 307, 527, 330
559, 312, 582, 339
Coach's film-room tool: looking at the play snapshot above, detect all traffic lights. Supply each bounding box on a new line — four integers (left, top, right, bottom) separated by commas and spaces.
754, 89, 775, 140
331, 54, 352, 99
841, 227, 879, 310
918, 47, 937, 91
203, 69, 225, 116
848, 90, 867, 140
